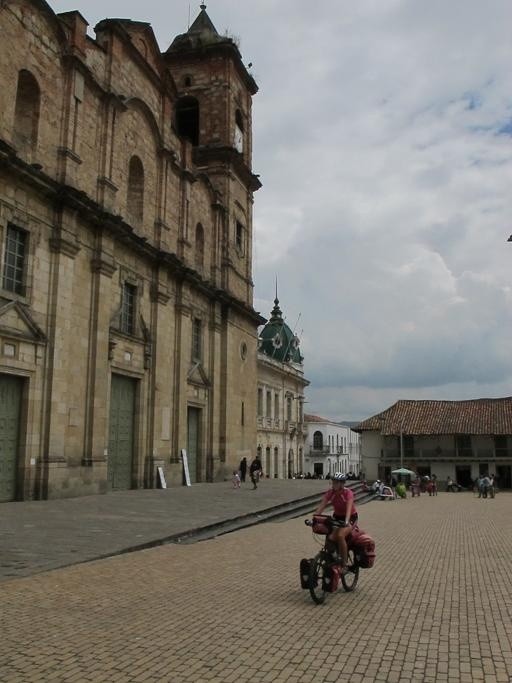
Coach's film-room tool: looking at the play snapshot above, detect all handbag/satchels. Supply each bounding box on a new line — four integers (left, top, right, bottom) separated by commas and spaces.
312, 514, 332, 534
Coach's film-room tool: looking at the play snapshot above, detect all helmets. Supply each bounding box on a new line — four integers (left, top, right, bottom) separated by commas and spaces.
331, 472, 348, 480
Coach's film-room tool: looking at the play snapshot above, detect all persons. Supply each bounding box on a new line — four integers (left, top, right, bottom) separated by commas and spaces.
239, 456, 247, 483
249, 465, 258, 489
300, 471, 358, 572
230, 471, 241, 489
357, 466, 499, 501
261, 469, 365, 479
252, 456, 262, 480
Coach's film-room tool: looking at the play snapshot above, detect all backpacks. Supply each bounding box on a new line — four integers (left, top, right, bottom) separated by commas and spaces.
322, 563, 342, 593
300, 558, 319, 588
356, 534, 376, 568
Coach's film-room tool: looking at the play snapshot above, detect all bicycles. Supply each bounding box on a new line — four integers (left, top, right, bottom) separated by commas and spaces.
305, 520, 359, 604
